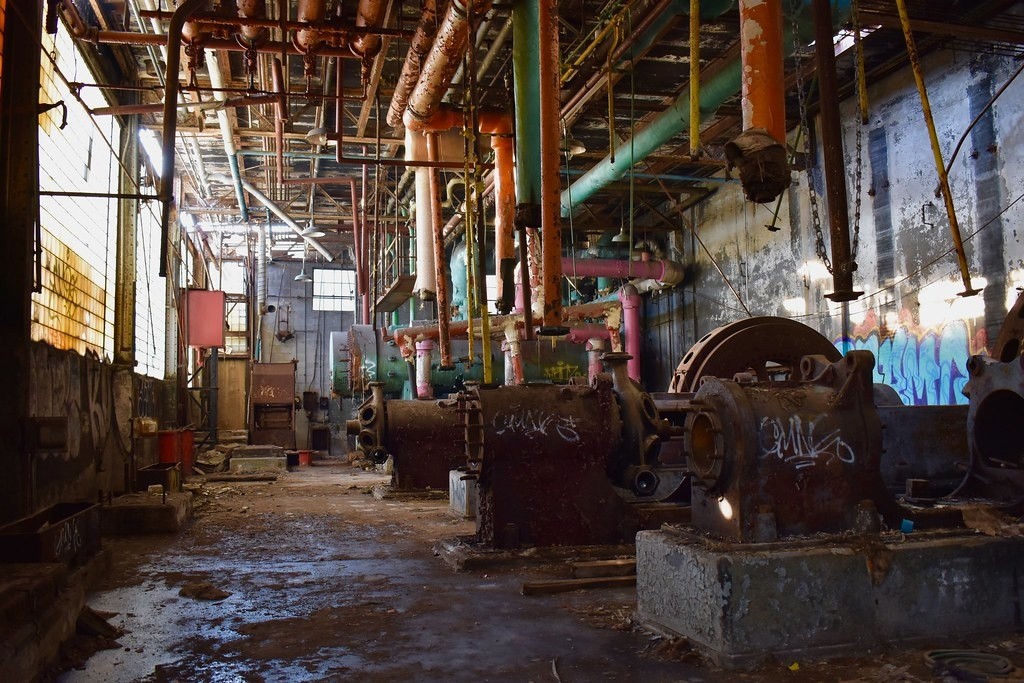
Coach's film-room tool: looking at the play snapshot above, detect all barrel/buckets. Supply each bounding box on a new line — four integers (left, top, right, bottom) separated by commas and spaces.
142, 420, 156, 433
158, 429, 195, 478
297, 450, 313, 466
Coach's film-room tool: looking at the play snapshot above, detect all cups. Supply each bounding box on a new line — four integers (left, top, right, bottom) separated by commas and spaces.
901, 519, 913, 533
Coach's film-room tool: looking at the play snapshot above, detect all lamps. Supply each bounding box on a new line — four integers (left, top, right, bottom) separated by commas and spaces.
560, 130, 586, 154
612, 198, 631, 241
305, 78, 334, 146
294, 244, 312, 281
301, 183, 325, 238
